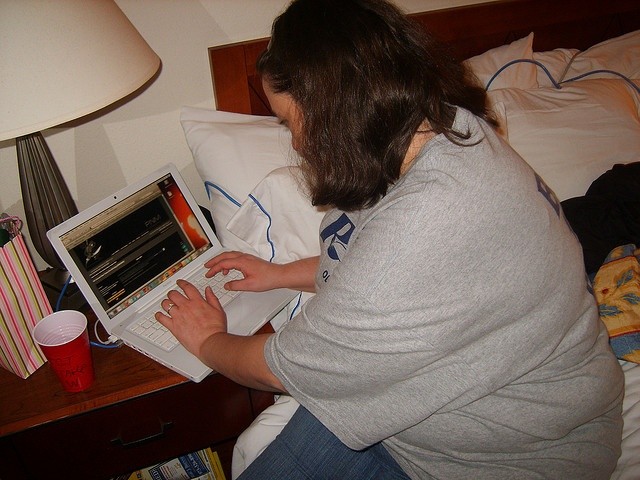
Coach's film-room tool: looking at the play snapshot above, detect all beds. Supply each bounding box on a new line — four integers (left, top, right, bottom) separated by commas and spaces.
208, 0, 640, 479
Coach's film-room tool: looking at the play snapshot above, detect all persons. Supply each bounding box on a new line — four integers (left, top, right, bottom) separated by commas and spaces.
158, 0, 626, 480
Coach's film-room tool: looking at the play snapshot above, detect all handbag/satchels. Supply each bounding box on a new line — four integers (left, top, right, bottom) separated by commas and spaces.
0, 213, 55, 379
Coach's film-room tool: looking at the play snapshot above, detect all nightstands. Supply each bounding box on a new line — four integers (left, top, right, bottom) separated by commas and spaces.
0, 230, 276, 480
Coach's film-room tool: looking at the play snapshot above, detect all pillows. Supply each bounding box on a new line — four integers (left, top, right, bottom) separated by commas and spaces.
534, 31, 640, 87
476, 77, 640, 254
179, 33, 538, 247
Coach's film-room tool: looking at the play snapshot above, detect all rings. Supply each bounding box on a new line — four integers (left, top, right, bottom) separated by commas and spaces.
166, 303, 175, 313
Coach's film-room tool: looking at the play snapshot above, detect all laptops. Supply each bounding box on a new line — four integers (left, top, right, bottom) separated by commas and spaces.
46, 163, 299, 384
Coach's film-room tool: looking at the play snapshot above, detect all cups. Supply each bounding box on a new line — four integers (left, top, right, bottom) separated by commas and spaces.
32, 308, 95, 395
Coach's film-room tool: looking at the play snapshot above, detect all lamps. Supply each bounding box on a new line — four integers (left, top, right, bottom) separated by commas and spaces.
0, 0, 163, 312
